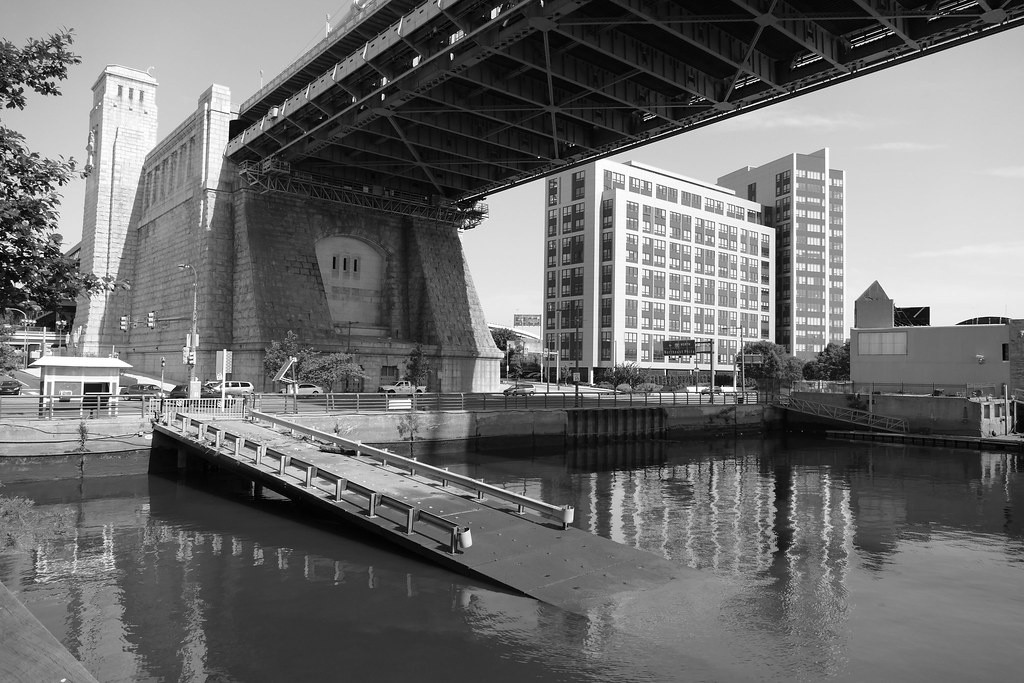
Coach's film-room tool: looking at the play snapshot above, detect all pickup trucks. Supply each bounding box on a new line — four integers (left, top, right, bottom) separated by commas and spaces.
376, 380, 428, 398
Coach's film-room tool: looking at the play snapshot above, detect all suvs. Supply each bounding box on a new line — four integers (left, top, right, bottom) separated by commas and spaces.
212, 381, 255, 398
503, 383, 537, 397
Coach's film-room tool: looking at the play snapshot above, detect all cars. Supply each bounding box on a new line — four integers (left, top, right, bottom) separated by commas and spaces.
121, 383, 170, 401
203, 381, 219, 389
702, 386, 722, 396
0, 379, 22, 395
508, 370, 542, 380
279, 384, 324, 398
170, 386, 222, 398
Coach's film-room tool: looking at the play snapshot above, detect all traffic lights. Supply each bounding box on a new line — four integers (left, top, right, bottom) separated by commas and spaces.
146, 311, 160, 332
187, 351, 197, 366
120, 315, 130, 332
161, 357, 166, 368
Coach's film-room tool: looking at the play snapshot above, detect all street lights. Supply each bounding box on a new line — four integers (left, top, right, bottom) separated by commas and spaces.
721, 325, 746, 405
347, 320, 360, 358
177, 263, 198, 399
5, 307, 26, 368
379, 338, 392, 384
56, 319, 67, 356
